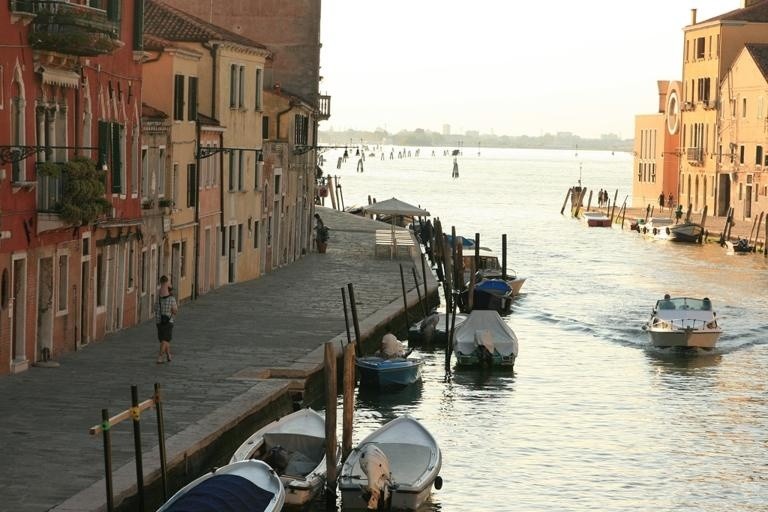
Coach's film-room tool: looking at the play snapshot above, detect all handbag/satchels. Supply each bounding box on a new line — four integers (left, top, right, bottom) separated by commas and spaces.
161, 315, 169, 325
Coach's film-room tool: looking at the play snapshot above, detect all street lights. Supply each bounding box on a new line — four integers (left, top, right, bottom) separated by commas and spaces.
0, 145, 109, 175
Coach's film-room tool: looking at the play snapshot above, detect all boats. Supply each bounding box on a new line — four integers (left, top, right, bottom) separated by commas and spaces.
724, 239, 754, 252
157, 459, 286, 511
669, 223, 703, 242
228, 405, 341, 507
355, 352, 426, 393
647, 296, 721, 350
583, 211, 611, 228
338, 415, 443, 509
354, 378, 424, 410
343, 198, 525, 367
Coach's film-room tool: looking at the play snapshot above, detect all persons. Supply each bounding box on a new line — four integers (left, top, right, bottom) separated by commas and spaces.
701, 298, 711, 310
159, 275, 172, 299
314, 213, 324, 250
598, 188, 608, 208
382, 334, 403, 358
660, 294, 675, 309
154, 296, 178, 364
420, 312, 439, 351
658, 191, 673, 212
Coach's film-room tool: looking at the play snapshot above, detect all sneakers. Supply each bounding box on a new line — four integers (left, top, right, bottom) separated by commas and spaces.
157, 354, 174, 363
